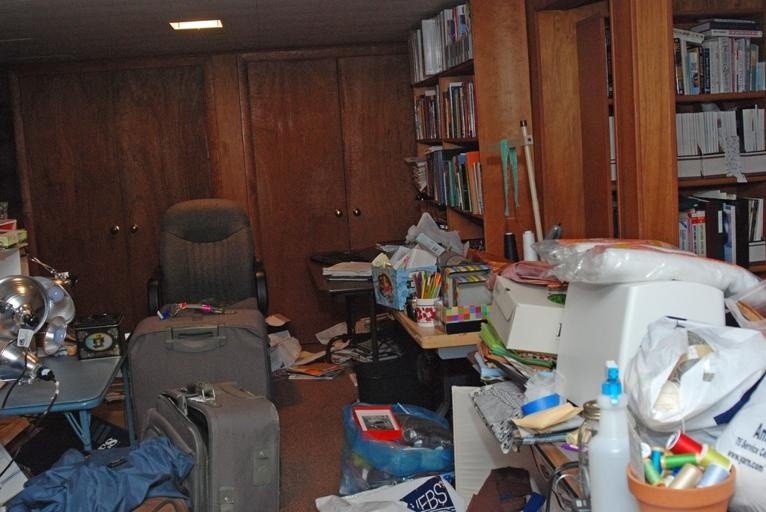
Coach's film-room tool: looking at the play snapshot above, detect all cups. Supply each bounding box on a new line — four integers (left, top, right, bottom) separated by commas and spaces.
406, 296, 417, 320
416, 298, 441, 329
627, 455, 736, 512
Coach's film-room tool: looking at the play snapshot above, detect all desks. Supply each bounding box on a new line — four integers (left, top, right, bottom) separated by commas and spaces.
303, 242, 412, 367
0, 330, 136, 460
389, 304, 492, 419
464, 337, 601, 512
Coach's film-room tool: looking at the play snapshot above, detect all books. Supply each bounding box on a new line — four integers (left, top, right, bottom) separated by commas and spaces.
404, 143, 483, 214
414, 82, 476, 138
679, 188, 766, 266
674, 103, 766, 178
329, 275, 369, 282
672, 19, 765, 96
322, 259, 373, 278
266, 313, 396, 379
409, 4, 473, 84
471, 322, 553, 381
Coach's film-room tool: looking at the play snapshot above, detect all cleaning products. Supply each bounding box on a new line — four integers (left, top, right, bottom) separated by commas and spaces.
589, 359, 640, 512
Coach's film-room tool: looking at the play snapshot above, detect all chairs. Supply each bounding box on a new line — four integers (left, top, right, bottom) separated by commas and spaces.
143, 196, 270, 322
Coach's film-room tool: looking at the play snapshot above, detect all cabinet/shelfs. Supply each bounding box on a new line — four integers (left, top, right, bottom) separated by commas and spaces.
13, 63, 212, 340
243, 51, 413, 349
410, 1, 535, 270
576, 9, 766, 273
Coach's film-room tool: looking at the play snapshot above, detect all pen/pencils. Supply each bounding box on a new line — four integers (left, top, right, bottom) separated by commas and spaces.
413, 269, 443, 299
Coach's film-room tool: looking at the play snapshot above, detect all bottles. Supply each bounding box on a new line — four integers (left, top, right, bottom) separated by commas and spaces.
577, 401, 604, 502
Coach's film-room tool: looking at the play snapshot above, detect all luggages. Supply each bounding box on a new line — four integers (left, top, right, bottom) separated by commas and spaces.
128, 303, 271, 440
141, 380, 279, 511
